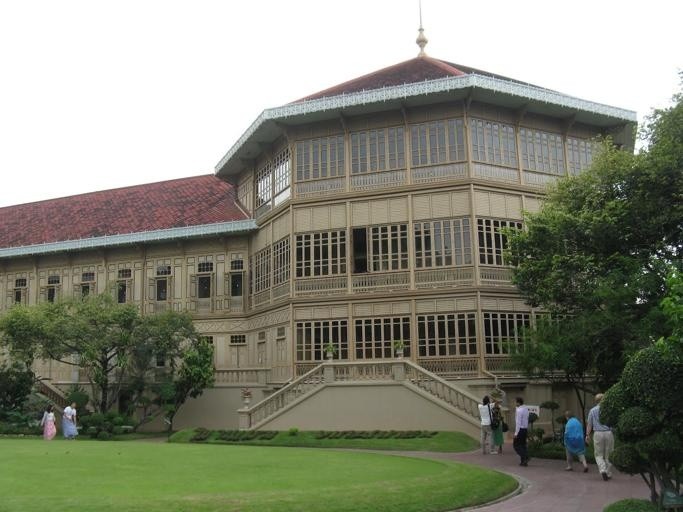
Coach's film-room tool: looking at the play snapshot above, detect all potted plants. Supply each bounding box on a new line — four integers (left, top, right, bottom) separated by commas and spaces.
324, 343, 337, 358
393, 341, 406, 357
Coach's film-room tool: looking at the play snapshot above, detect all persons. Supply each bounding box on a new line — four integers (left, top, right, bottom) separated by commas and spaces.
477, 395, 500, 456
491, 399, 504, 454
41, 405, 57, 440
562, 409, 589, 473
512, 396, 530, 467
584, 393, 616, 481
61, 401, 78, 440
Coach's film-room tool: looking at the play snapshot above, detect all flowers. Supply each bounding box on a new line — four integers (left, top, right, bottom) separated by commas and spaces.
239, 385, 251, 396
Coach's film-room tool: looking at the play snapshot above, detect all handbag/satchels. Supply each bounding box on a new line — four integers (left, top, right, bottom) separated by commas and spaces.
491, 418, 499, 430
502, 423, 509, 432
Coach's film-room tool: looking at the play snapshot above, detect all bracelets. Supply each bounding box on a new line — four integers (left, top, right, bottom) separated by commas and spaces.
586, 433, 590, 436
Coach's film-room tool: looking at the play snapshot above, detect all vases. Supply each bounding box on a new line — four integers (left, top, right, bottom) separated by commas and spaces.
243, 396, 250, 409
